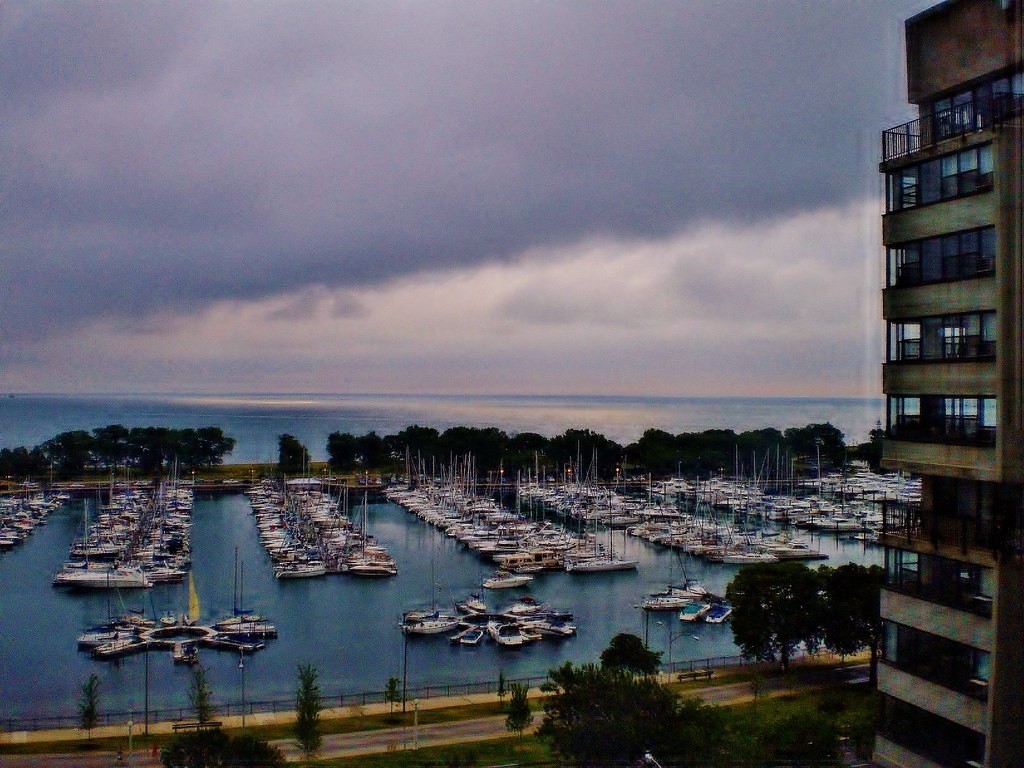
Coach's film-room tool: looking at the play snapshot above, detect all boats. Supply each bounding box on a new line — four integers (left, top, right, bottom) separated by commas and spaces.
2, 453, 921, 667
